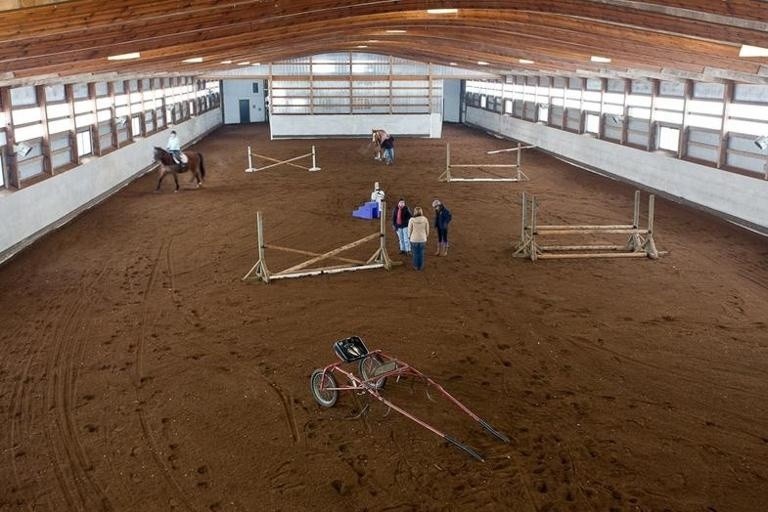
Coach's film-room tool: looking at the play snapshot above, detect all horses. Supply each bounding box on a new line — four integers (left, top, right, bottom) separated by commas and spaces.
371, 128, 388, 162
151, 146, 205, 193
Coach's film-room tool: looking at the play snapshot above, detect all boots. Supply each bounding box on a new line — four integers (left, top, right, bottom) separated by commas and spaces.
432, 200, 441, 206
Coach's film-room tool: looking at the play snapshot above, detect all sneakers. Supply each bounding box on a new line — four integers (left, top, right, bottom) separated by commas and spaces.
398, 250, 412, 256
434, 244, 447, 257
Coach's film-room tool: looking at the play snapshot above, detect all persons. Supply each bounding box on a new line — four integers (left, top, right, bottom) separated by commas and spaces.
380, 133, 394, 166
391, 198, 452, 272
166, 130, 188, 164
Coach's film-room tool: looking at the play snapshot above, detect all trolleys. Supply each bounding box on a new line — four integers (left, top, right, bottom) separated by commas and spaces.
311, 331, 511, 470
